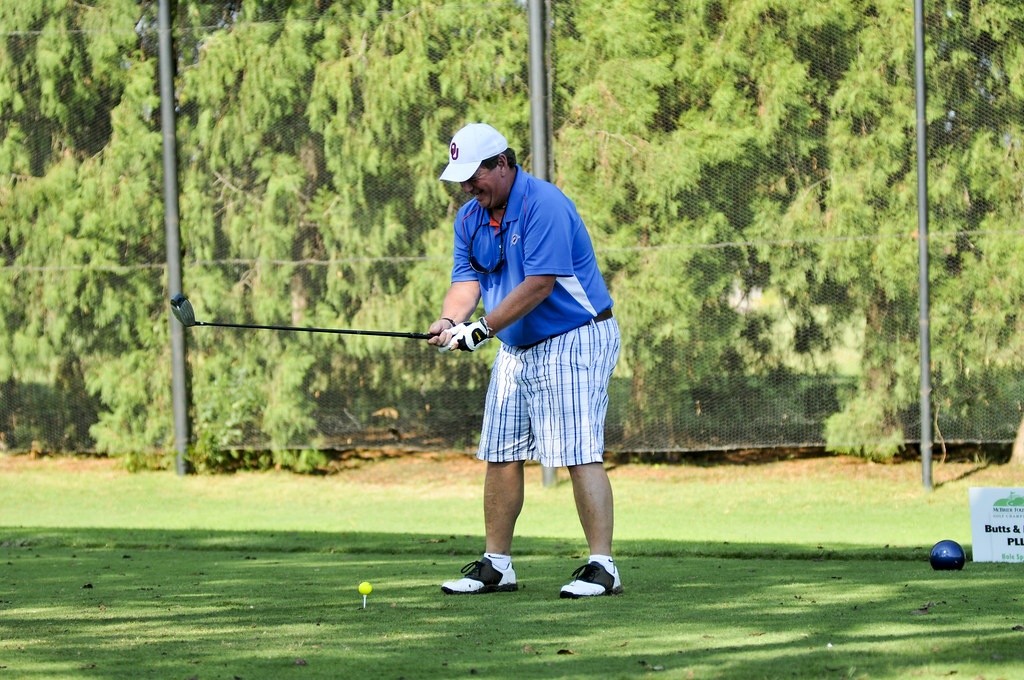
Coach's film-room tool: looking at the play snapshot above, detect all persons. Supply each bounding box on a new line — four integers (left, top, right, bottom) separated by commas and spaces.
428, 125, 624, 598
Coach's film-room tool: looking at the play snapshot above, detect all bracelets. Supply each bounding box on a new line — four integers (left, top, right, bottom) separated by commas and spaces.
442, 317, 456, 328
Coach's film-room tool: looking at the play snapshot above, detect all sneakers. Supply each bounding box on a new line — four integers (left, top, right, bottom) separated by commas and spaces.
440, 557, 518, 595
559, 561, 625, 598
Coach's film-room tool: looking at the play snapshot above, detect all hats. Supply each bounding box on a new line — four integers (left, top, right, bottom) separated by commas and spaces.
438, 123, 509, 183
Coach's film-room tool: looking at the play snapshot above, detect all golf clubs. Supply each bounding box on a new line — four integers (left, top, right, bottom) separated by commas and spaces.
169, 292, 441, 339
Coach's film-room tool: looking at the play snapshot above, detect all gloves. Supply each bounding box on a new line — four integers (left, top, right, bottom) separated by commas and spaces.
434, 317, 490, 353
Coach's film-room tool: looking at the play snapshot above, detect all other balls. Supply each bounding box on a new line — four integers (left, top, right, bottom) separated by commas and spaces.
930, 539, 966, 571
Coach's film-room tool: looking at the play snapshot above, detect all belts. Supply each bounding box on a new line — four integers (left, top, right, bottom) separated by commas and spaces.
518, 307, 614, 350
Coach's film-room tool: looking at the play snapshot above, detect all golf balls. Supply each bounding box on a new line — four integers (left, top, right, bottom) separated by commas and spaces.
358, 582, 372, 595
